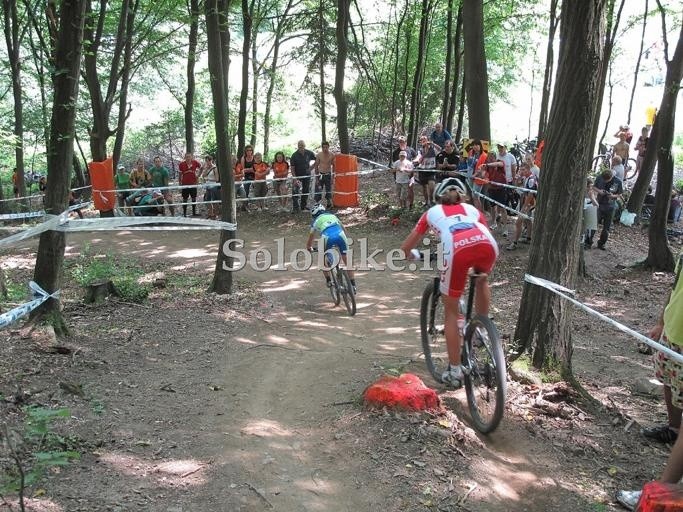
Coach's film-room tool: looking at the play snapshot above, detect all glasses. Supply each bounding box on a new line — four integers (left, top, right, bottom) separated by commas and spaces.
39, 175, 44, 178
497, 145, 503, 149
245, 149, 251, 151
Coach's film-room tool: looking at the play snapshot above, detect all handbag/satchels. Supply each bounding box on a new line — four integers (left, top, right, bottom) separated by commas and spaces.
506, 193, 522, 215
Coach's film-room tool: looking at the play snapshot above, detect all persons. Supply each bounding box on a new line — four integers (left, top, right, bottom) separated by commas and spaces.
3, 164, 84, 220
614, 411, 683, 512
307, 203, 356, 294
638, 245, 682, 444
400, 176, 499, 391
112, 140, 335, 218
388, 101, 656, 251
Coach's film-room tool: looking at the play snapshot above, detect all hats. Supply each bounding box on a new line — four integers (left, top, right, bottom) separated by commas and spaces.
399, 151, 407, 157
39, 175, 45, 179
397, 136, 407, 143
495, 142, 508, 147
116, 163, 125, 171
153, 189, 162, 196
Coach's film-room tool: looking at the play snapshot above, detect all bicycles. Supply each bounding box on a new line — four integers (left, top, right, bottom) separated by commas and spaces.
507, 135, 538, 174
409, 248, 507, 435
590, 143, 638, 179
304, 247, 357, 316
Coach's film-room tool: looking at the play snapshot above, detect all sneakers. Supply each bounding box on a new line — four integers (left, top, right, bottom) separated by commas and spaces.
345, 286, 356, 294
326, 280, 332, 287
280, 205, 291, 213
583, 242, 592, 250
519, 238, 530, 244
261, 206, 270, 211
640, 425, 678, 443
597, 240, 606, 250
327, 204, 333, 211
489, 223, 498, 230
472, 326, 488, 348
506, 242, 519, 250
257, 207, 263, 212
615, 489, 643, 512
302, 207, 311, 213
441, 370, 462, 388
292, 208, 299, 214
502, 232, 509, 239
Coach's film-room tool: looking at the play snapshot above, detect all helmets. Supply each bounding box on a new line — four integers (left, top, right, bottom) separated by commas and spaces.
311, 203, 326, 218
432, 177, 468, 204
